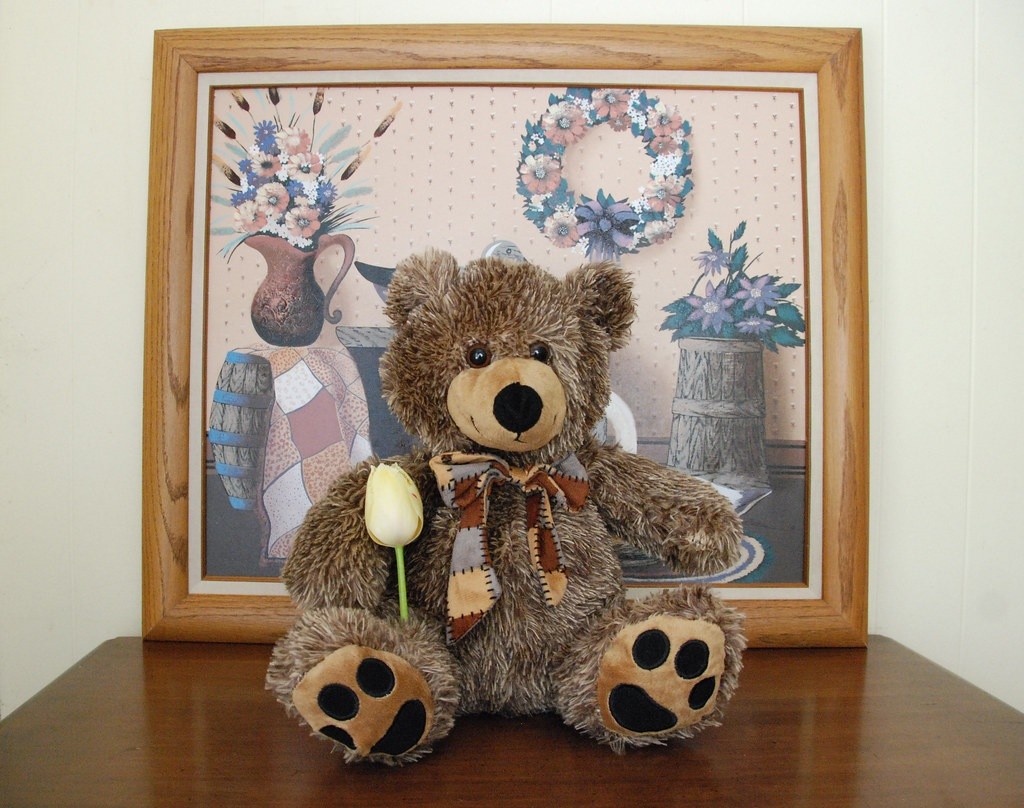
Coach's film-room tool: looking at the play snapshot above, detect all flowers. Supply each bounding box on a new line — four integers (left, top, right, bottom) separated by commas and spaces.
657, 220, 808, 355
211, 86, 401, 254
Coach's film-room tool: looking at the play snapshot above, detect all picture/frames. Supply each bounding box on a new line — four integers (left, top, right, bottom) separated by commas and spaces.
140, 25, 869, 652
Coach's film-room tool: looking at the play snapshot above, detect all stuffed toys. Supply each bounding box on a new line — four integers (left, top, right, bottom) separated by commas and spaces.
263, 247, 745, 769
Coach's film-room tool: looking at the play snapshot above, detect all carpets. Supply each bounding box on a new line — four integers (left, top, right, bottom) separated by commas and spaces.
607, 525, 776, 587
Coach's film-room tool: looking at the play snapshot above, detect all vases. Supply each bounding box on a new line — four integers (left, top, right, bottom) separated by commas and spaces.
666, 334, 770, 489
243, 232, 354, 347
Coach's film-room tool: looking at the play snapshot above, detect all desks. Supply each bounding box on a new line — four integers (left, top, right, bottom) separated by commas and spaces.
0, 633, 1023, 808
336, 327, 409, 458
